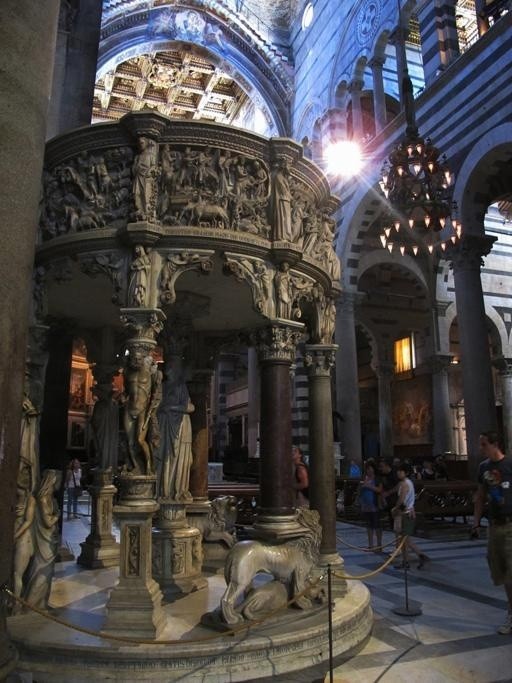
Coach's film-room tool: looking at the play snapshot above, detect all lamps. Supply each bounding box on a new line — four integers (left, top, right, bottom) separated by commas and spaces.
376, 0, 464, 257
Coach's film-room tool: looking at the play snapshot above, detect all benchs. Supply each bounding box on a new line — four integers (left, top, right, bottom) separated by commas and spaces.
337, 473, 481, 524
208, 480, 261, 519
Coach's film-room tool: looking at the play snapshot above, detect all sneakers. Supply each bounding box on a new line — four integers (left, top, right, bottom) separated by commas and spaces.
496, 610, 512, 634
417, 554, 431, 569
393, 560, 410, 569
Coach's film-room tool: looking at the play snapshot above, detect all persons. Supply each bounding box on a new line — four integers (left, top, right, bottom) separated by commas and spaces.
35, 133, 343, 282
392, 465, 430, 570
128, 245, 150, 308
348, 458, 461, 500
325, 299, 337, 343
290, 448, 309, 506
120, 347, 164, 478
469, 431, 512, 635
66, 458, 83, 521
359, 463, 385, 552
274, 262, 293, 319
160, 353, 195, 500
90, 386, 117, 471
11, 381, 64, 617
